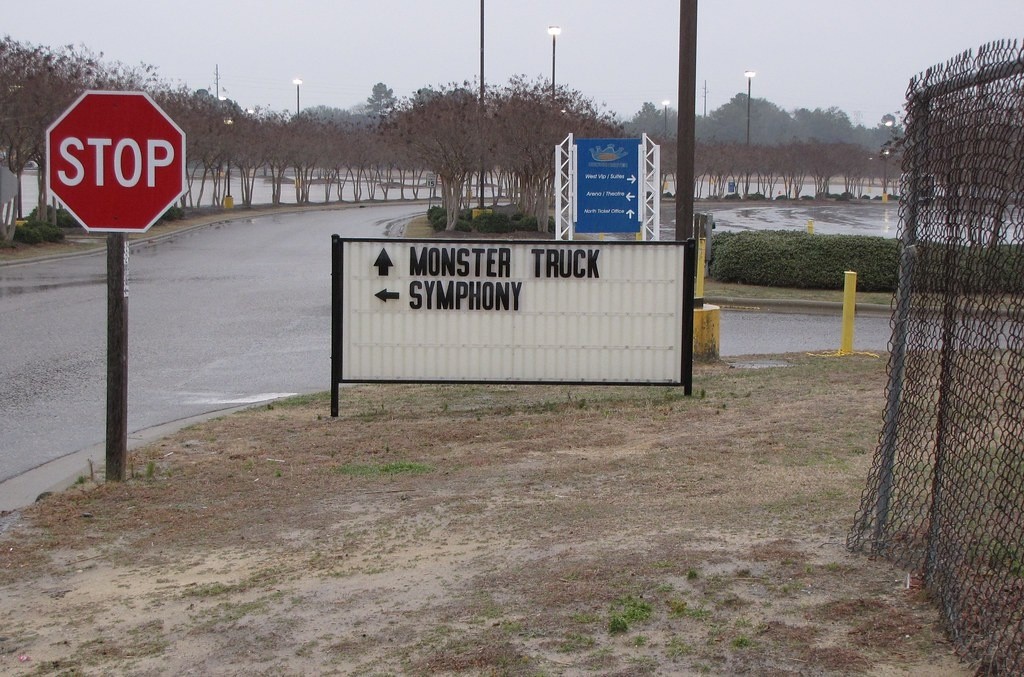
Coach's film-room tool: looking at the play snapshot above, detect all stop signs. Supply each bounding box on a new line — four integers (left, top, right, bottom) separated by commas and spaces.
45, 91, 189, 232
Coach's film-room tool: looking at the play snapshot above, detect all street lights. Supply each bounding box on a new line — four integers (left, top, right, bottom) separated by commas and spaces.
223, 114, 234, 208
662, 100, 670, 192
745, 71, 756, 143
882, 149, 890, 204
867, 157, 872, 193
293, 78, 303, 187
548, 25, 561, 102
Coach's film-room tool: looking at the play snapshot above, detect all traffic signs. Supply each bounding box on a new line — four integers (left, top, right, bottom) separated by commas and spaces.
574, 139, 643, 234
